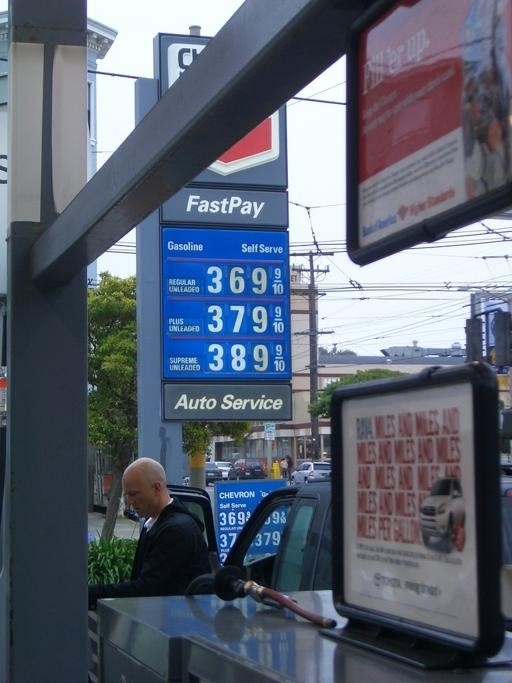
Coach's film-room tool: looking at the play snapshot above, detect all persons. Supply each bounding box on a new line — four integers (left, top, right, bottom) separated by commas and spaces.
279, 457, 288, 478
121, 456, 211, 597
285, 455, 293, 481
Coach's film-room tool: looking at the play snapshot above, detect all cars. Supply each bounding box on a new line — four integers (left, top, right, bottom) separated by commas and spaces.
204, 457, 265, 484
164, 480, 336, 595
420, 479, 468, 550
498, 462, 512, 498
289, 460, 332, 485
